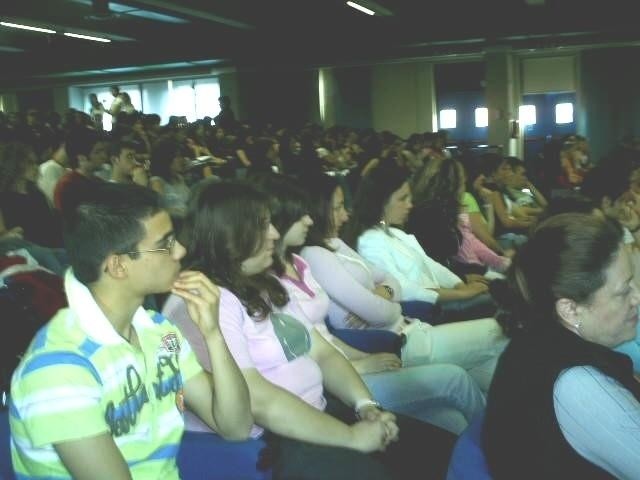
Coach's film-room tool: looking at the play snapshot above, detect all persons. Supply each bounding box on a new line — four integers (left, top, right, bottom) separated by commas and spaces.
1, 86, 640, 480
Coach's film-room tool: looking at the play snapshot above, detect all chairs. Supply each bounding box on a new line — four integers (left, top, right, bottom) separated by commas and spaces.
0, 171, 96, 389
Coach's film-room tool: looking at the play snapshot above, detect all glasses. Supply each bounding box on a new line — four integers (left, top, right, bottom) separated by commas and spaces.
103, 232, 177, 273
